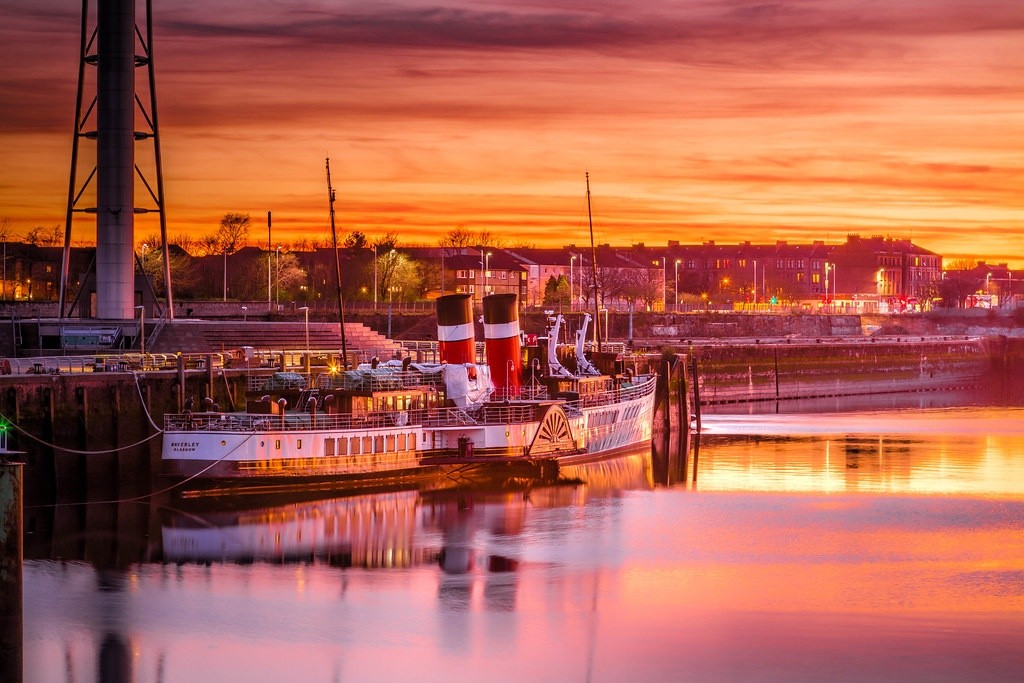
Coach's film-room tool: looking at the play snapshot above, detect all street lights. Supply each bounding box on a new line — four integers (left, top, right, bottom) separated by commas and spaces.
579, 252, 582, 315
142, 242, 148, 268
762, 267, 765, 296
571, 256, 576, 314
134, 305, 144, 354
299, 306, 309, 355
660, 256, 666, 314
675, 259, 682, 314
486, 252, 492, 297
275, 245, 282, 319
753, 260, 757, 301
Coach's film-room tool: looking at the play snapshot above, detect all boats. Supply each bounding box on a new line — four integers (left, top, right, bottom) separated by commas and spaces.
154, 152, 702, 480
